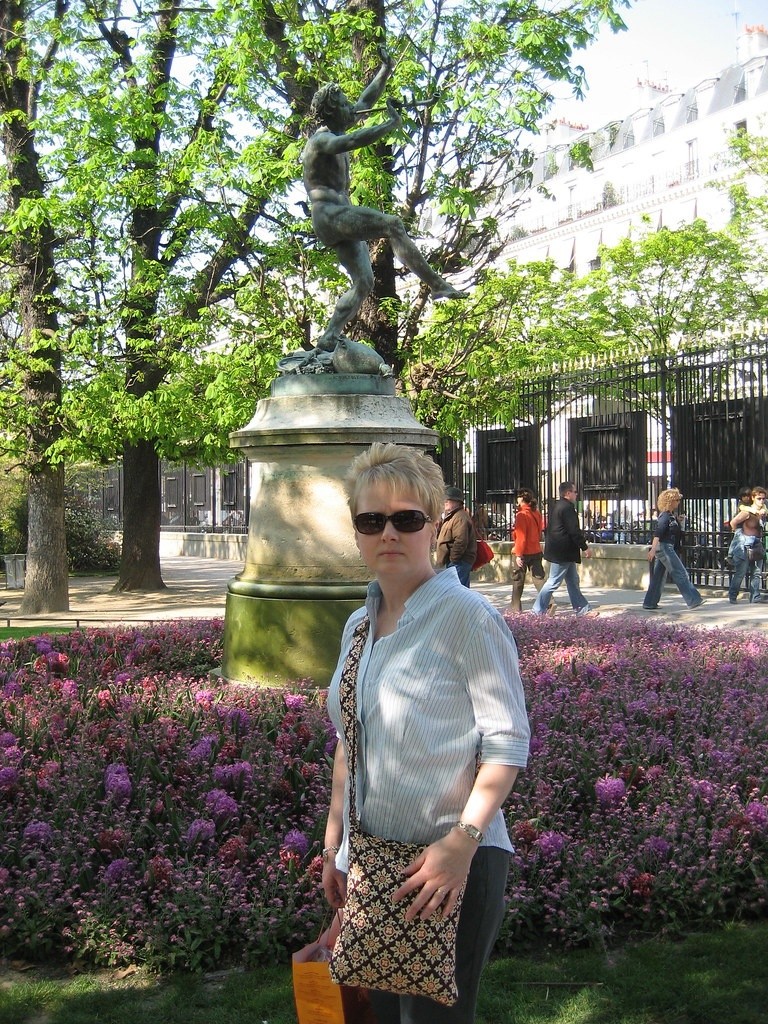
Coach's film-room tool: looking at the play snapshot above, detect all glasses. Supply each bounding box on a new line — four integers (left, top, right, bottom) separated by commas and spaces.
571, 491, 577, 494
516, 494, 523, 499
354, 510, 431, 535
755, 498, 765, 501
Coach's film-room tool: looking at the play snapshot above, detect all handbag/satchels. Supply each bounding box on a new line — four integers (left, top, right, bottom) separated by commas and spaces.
747, 541, 764, 562
488, 516, 493, 534
292, 897, 375, 1024
471, 540, 494, 570
329, 827, 468, 1007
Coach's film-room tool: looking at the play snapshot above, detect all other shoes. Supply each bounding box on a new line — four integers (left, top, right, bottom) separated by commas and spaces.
643, 605, 662, 609
547, 604, 557, 617
688, 599, 707, 609
579, 611, 600, 618
730, 598, 737, 603
727, 557, 734, 566
749, 599, 761, 603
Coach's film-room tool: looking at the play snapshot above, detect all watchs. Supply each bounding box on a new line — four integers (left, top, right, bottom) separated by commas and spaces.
455, 820, 483, 842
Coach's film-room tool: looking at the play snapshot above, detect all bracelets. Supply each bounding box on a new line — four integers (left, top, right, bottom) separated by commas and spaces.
322, 846, 340, 862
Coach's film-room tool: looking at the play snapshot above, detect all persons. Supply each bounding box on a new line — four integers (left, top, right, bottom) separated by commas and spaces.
321, 444, 532, 1024
300, 43, 471, 350
434, 480, 768, 621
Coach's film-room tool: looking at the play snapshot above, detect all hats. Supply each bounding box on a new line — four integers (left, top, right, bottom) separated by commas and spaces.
472, 497, 483, 504
445, 487, 463, 501
